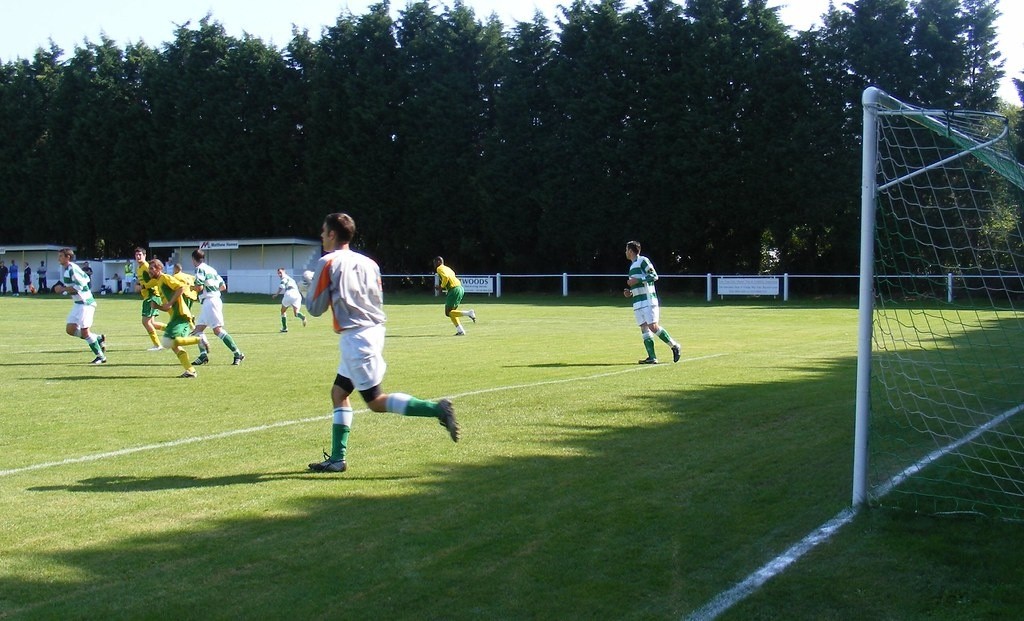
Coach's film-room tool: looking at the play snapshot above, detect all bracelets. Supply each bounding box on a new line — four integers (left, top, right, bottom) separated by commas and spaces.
629, 289, 632, 296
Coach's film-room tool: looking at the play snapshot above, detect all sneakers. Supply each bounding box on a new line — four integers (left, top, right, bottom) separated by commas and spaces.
230, 353, 245, 365
191, 356, 208, 365
438, 399, 460, 443
671, 343, 681, 362
638, 357, 658, 364
308, 458, 346, 472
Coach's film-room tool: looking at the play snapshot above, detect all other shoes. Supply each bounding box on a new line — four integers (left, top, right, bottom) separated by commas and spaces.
195, 332, 209, 353
301, 315, 306, 327
98, 335, 106, 353
280, 329, 287, 332
469, 310, 476, 323
453, 331, 465, 336
147, 346, 163, 351
176, 370, 197, 379
88, 357, 106, 365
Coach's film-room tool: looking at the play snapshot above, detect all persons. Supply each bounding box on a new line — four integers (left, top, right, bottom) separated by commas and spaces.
306, 213, 461, 473
0, 261, 8, 295
37, 260, 47, 294
624, 240, 681, 364
125, 259, 133, 294
273, 267, 306, 332
134, 246, 209, 378
9, 260, 20, 296
434, 256, 476, 336
55, 249, 107, 365
189, 249, 245, 365
23, 262, 32, 295
109, 273, 120, 289
82, 262, 93, 289
